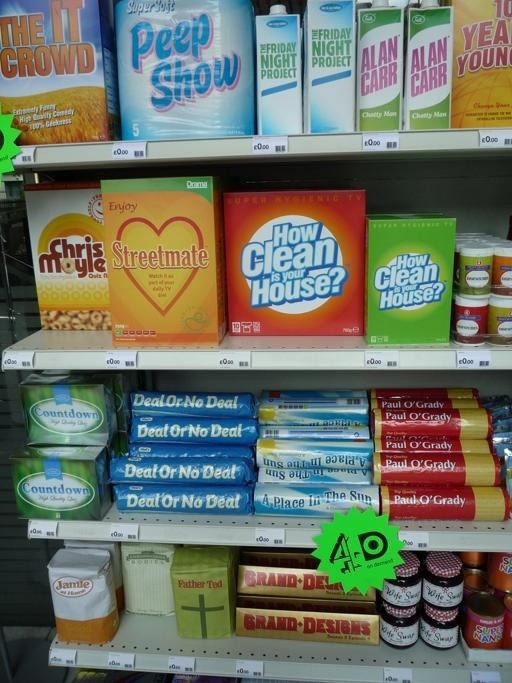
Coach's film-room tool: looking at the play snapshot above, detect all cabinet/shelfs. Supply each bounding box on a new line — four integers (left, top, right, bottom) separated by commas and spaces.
1, 0, 512, 682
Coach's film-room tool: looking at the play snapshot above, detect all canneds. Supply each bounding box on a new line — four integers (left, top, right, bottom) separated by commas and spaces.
379, 552, 512, 651
